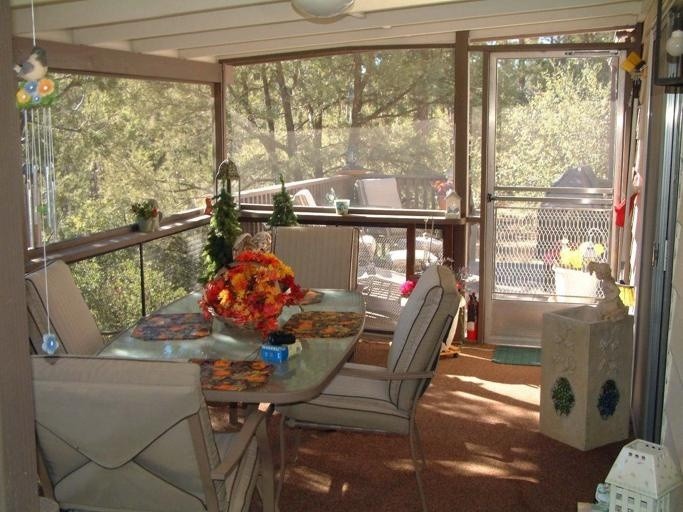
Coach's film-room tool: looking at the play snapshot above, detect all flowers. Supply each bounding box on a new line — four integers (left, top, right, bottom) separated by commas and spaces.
540, 235, 606, 277
199, 249, 306, 335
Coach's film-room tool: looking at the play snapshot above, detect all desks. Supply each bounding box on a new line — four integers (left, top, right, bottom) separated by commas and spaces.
94, 287, 366, 509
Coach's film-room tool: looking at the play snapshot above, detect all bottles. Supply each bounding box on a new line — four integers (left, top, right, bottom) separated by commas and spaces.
466, 293, 478, 341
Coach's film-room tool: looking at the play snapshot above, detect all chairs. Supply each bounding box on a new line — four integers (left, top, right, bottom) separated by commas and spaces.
26, 258, 119, 352
269, 225, 359, 290
28, 354, 274, 509
273, 258, 458, 511
287, 174, 481, 312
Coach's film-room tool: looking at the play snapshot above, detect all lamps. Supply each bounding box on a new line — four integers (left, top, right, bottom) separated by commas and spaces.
293, 1, 353, 19
580, 227, 609, 268
666, 20, 683, 56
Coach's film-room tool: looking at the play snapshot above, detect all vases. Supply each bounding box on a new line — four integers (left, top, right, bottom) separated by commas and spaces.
210, 307, 282, 332
549, 265, 598, 308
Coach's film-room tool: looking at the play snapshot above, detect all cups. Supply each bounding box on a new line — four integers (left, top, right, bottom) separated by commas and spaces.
334, 199, 349, 215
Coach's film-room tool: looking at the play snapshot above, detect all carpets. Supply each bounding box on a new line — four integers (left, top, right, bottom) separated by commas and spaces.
491, 346, 540, 365
